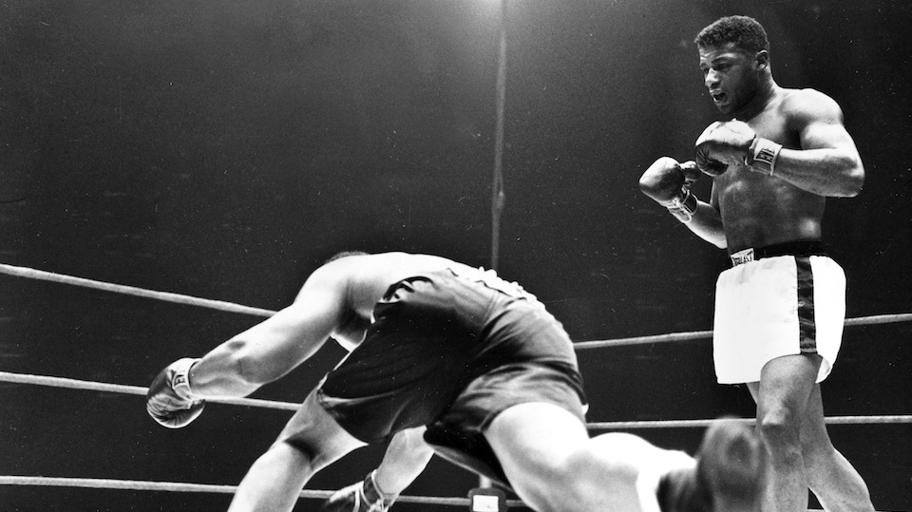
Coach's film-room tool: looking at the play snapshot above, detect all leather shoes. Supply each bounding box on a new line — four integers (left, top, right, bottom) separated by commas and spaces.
695, 120, 782, 179
638, 156, 699, 222
317, 469, 399, 512
146, 355, 205, 430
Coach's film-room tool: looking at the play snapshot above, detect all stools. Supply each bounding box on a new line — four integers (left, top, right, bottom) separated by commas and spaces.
723, 241, 833, 268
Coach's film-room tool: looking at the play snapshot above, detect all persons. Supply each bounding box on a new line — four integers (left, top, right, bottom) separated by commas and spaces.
144, 247, 773, 512
635, 14, 878, 512
